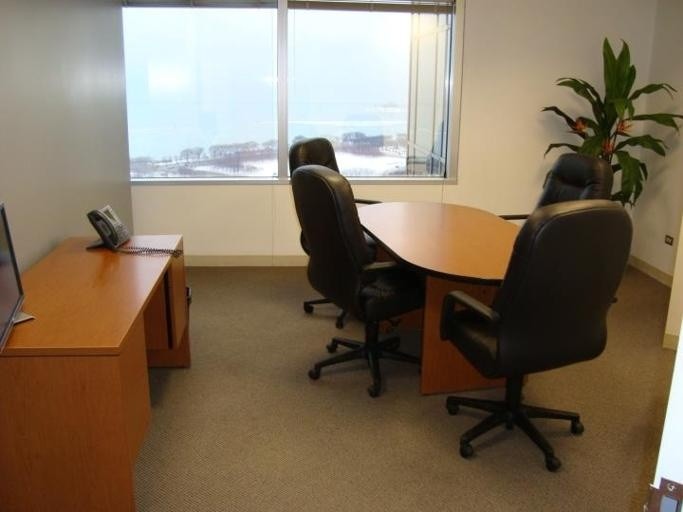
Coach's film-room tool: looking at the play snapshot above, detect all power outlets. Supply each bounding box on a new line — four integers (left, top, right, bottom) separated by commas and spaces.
664, 234, 673, 246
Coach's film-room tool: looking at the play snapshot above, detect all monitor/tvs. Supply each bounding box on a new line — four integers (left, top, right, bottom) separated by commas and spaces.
0, 204, 24, 359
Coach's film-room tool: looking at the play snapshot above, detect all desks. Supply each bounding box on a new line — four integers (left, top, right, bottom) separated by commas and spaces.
358, 202, 546, 396
0, 235, 191, 511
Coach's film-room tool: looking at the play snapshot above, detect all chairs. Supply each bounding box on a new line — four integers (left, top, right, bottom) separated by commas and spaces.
291, 164, 424, 398
438, 199, 632, 473
288, 138, 383, 247
498, 152, 613, 221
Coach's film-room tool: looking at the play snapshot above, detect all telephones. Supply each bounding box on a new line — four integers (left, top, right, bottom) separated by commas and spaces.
87, 205, 130, 252
